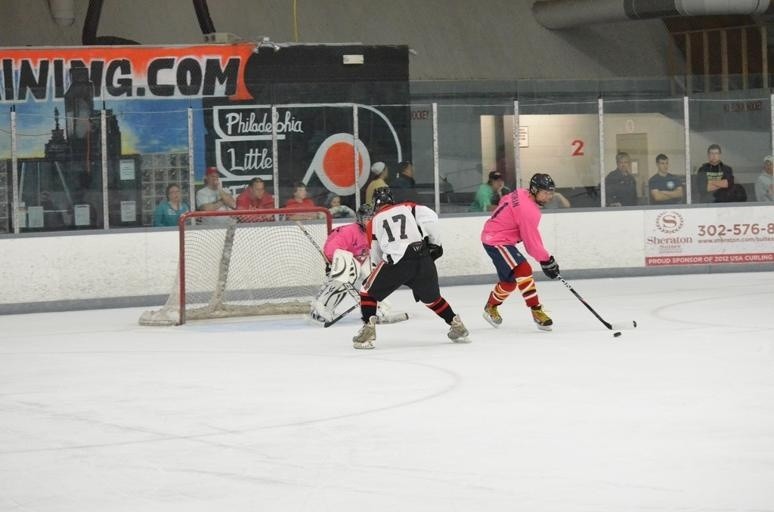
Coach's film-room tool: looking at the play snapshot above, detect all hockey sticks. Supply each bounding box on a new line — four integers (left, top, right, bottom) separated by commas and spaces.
324, 302, 360, 327
295, 220, 408, 323
556, 273, 637, 331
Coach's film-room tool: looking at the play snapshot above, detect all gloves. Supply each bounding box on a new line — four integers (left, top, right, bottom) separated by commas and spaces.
540, 256, 560, 279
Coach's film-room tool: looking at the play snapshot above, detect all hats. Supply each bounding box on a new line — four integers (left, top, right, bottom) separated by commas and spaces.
487, 170, 502, 184
371, 162, 385, 180
764, 155, 774, 164
206, 166, 219, 175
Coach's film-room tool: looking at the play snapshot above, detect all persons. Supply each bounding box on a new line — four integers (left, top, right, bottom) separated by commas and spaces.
391, 161, 418, 205
236, 177, 274, 222
284, 182, 315, 217
696, 144, 734, 203
364, 162, 391, 208
605, 152, 638, 207
540, 192, 571, 208
754, 155, 773, 203
649, 154, 684, 205
195, 167, 236, 223
468, 171, 512, 212
481, 173, 560, 330
353, 186, 471, 349
312, 204, 373, 325
152, 182, 190, 228
325, 193, 345, 218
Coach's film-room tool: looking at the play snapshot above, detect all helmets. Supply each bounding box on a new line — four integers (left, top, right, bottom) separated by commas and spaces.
530, 174, 555, 206
355, 187, 395, 228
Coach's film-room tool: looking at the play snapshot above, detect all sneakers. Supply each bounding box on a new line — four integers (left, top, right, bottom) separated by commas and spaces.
484, 303, 502, 324
531, 305, 552, 326
448, 314, 468, 339
353, 316, 376, 343
